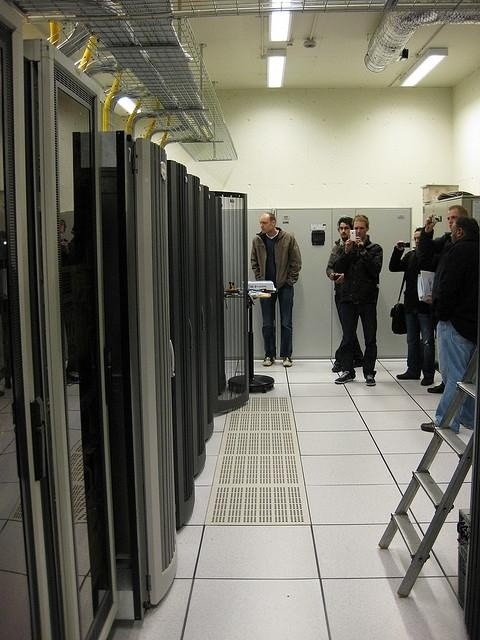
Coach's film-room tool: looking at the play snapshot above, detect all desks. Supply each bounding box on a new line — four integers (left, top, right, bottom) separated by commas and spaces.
226, 290, 278, 394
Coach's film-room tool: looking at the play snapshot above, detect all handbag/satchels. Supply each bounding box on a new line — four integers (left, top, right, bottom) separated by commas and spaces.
390, 303, 407, 334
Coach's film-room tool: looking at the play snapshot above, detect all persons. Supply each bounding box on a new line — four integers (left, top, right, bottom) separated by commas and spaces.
421, 205, 468, 393
389, 228, 435, 386
251, 212, 302, 367
60, 220, 79, 386
421, 216, 479, 434
326, 217, 364, 372
335, 215, 384, 386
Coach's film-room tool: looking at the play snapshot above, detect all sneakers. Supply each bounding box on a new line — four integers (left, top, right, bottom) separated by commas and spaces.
283, 356, 293, 367
366, 371, 376, 386
335, 370, 353, 384
263, 356, 276, 366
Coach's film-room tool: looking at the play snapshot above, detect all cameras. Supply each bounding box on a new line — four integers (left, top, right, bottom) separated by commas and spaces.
397, 242, 410, 250
432, 215, 442, 223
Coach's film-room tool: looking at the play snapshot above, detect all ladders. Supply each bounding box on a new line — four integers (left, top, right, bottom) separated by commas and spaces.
378, 346, 480, 598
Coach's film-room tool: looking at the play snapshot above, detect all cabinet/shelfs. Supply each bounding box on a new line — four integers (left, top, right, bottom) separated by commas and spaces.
221, 206, 413, 363
422, 193, 480, 244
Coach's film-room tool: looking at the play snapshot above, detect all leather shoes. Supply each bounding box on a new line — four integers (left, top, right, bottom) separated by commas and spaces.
397, 371, 411, 379
428, 383, 443, 393
421, 422, 435, 432
353, 359, 363, 367
332, 360, 342, 372
421, 378, 434, 386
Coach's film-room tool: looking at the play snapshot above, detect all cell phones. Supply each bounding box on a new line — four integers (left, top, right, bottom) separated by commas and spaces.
349, 230, 357, 242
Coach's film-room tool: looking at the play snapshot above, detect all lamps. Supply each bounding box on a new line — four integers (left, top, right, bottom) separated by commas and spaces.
398, 47, 449, 88
266, 50, 287, 90
267, 10, 292, 52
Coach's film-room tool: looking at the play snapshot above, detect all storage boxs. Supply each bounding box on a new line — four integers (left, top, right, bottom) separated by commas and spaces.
419, 183, 459, 205
